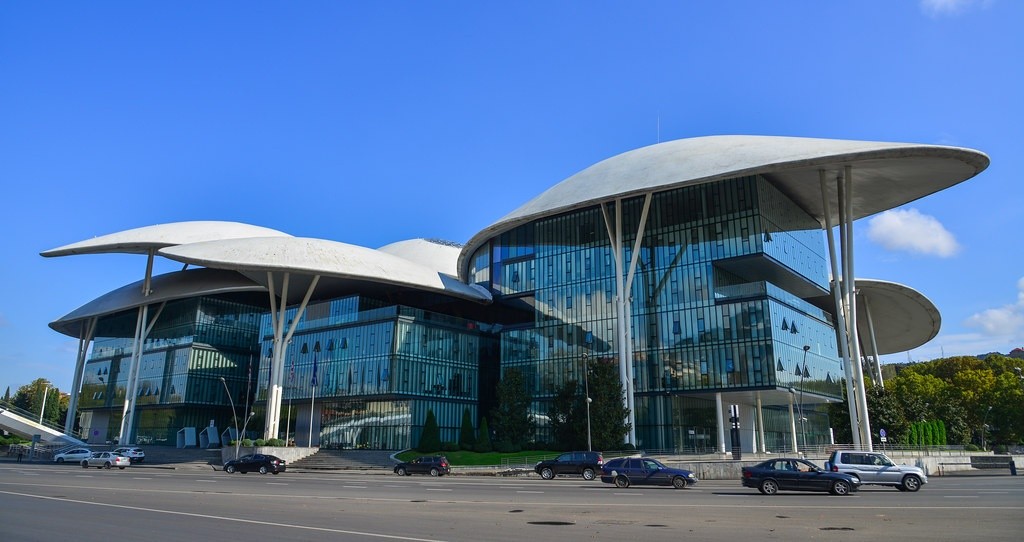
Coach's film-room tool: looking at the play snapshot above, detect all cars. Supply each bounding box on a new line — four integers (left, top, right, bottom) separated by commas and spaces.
53, 448, 99, 464
223, 453, 286, 475
80, 451, 131, 469
740, 457, 861, 496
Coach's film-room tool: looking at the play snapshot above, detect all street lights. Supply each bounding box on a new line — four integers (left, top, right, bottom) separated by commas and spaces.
581, 352, 593, 452
220, 375, 255, 460
788, 344, 811, 458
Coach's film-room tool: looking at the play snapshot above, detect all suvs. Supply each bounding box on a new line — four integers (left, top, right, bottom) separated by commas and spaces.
600, 456, 699, 489
533, 450, 604, 480
392, 454, 451, 478
823, 449, 928, 493
106, 446, 146, 463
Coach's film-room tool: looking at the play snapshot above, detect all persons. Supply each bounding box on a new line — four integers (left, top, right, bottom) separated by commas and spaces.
16, 446, 23, 464
